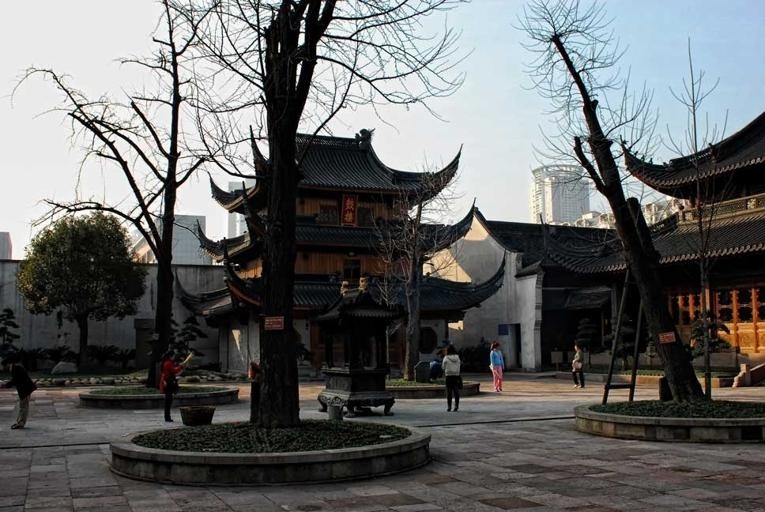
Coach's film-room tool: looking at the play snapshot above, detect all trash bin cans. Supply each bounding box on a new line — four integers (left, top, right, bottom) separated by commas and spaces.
414, 361, 430, 383
659, 376, 673, 402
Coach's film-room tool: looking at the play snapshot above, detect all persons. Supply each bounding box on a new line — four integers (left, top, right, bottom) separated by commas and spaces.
0, 358, 37, 429
159, 350, 186, 422
489, 340, 505, 392
430, 357, 443, 380
571, 343, 585, 388
441, 344, 461, 412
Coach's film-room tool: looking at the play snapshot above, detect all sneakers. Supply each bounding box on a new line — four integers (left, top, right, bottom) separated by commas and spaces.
494, 388, 503, 392
446, 407, 458, 412
572, 385, 585, 389
10, 423, 26, 430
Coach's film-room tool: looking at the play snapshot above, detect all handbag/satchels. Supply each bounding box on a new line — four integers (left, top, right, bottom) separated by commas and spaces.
457, 374, 465, 391
166, 374, 179, 395
571, 360, 584, 371
